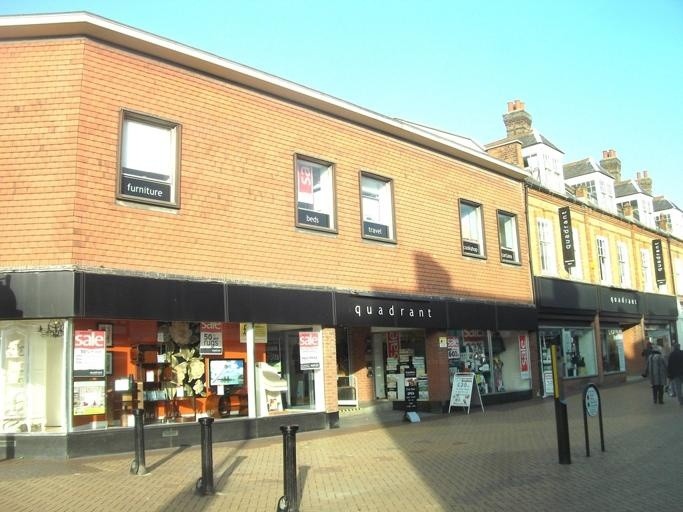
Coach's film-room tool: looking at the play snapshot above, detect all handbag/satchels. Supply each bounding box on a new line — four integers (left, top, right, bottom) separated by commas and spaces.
666, 385, 677, 397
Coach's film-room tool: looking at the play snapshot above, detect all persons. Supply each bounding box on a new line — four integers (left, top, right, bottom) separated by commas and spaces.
668, 344, 683, 406
641, 345, 668, 404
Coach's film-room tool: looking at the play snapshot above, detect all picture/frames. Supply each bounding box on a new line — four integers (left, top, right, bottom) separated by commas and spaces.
99, 323, 113, 376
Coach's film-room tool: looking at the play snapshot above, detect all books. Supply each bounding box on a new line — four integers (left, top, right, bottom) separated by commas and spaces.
386, 348, 429, 399
